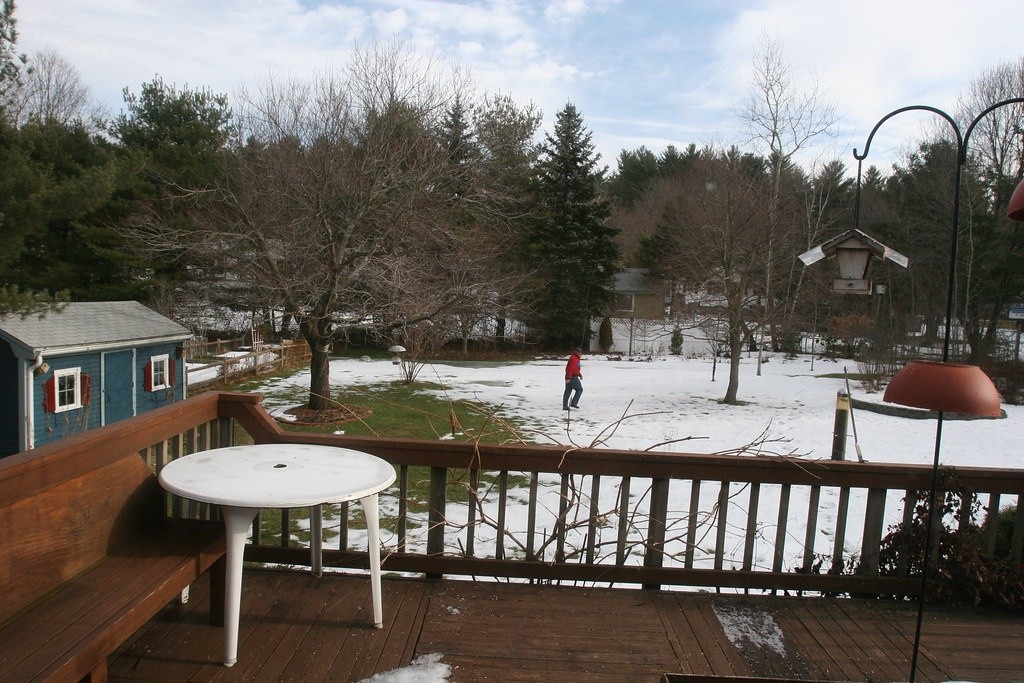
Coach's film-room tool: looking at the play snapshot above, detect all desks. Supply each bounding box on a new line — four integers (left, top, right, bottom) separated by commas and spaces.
156, 442, 397, 670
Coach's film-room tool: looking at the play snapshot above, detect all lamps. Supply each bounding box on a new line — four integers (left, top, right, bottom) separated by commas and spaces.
791, 157, 913, 297
387, 345, 406, 365
1006, 179, 1023, 224
883, 361, 1001, 421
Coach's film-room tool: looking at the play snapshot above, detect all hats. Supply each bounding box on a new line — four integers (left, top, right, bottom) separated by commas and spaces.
574, 347, 583, 352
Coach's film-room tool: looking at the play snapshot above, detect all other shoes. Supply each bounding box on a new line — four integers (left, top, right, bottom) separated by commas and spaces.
563, 407, 571, 410
570, 404, 579, 409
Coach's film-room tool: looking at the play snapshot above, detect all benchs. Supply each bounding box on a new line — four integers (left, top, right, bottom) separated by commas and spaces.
0, 452, 226, 682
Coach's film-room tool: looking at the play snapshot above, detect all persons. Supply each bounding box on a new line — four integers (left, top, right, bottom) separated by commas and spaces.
563, 347, 583, 411
